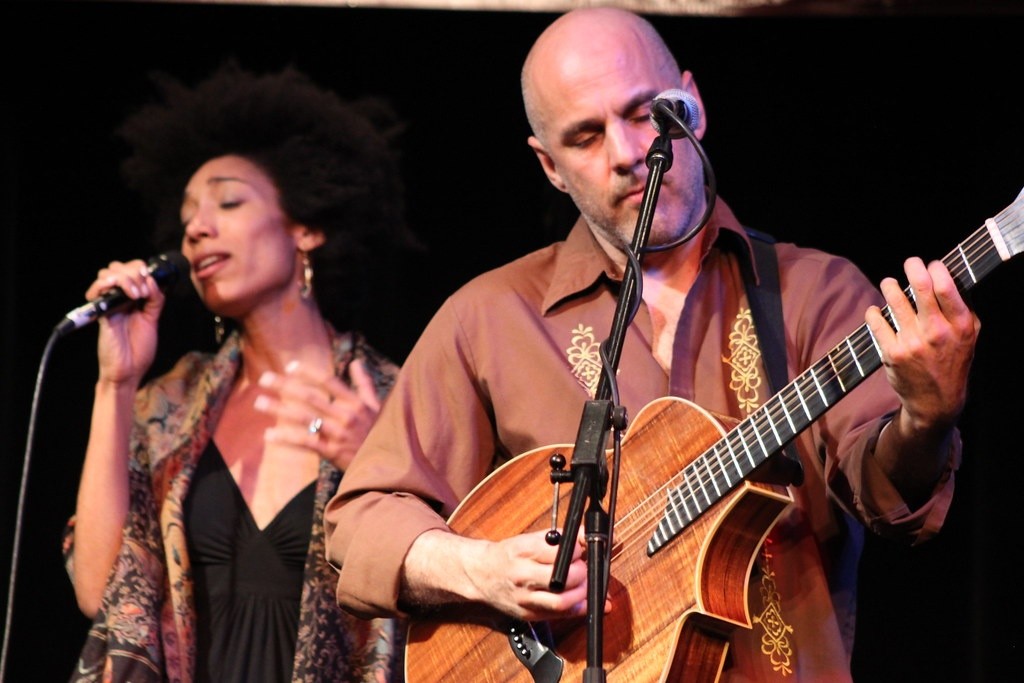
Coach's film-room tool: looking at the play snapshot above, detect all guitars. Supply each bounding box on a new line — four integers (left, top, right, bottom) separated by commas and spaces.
401, 188, 1024, 683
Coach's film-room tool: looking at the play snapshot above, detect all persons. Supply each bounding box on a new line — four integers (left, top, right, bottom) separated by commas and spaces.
61, 62, 428, 683
322, 8, 982, 683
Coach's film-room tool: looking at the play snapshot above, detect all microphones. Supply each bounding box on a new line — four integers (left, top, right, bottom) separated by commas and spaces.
55, 251, 190, 333
649, 88, 699, 137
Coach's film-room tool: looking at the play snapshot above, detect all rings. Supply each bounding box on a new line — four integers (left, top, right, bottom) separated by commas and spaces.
309, 419, 322, 432
